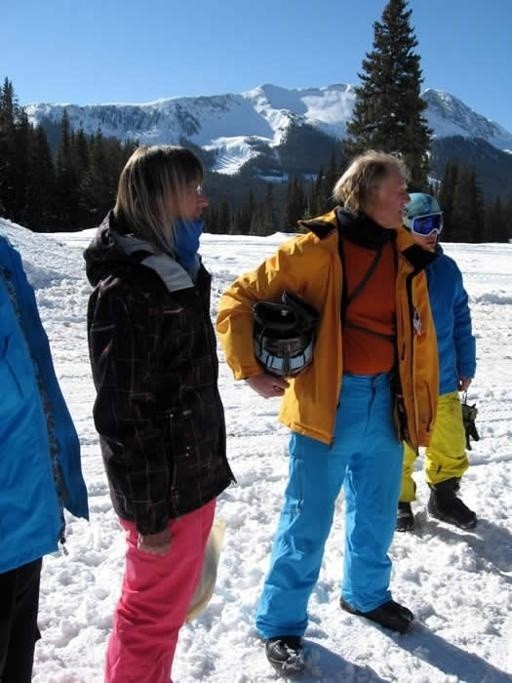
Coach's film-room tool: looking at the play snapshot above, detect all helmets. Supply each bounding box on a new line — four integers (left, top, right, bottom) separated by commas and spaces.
250, 290, 320, 379
401, 192, 444, 237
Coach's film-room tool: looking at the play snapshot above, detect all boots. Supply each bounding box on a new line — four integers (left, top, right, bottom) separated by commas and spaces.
265, 634, 305, 674
339, 597, 415, 632
395, 502, 415, 533
427, 476, 478, 531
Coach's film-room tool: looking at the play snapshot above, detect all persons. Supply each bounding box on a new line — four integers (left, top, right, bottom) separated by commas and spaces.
392, 190, 479, 533
79, 143, 239, 681
216, 146, 441, 674
0, 233, 90, 682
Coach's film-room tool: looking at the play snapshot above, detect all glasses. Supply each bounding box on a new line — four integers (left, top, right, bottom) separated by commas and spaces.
405, 212, 444, 236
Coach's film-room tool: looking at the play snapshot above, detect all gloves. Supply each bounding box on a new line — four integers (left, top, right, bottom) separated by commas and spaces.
463, 403, 478, 451
